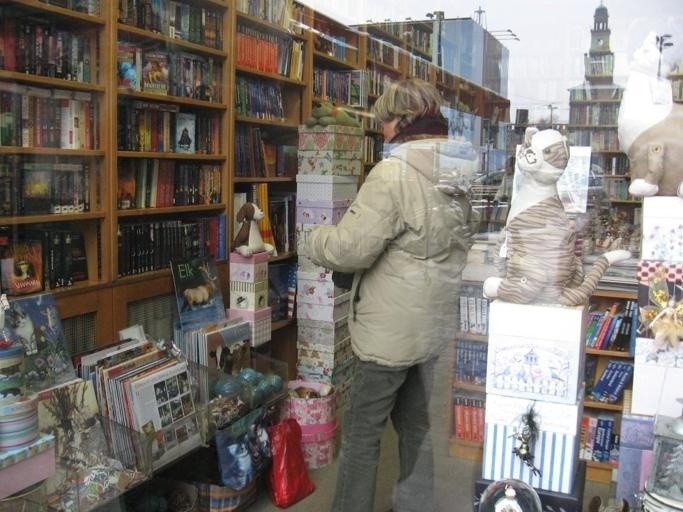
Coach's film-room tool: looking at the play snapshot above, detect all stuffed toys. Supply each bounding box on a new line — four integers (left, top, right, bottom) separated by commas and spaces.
476, 123, 634, 306
308, 101, 364, 128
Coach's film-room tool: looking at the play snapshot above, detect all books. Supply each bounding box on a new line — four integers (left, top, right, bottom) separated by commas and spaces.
445, 283, 638, 467
362, 39, 432, 186
116, 2, 228, 278
1, 294, 203, 478
358, 114, 384, 165
314, 13, 385, 112
169, 259, 252, 398
434, 68, 511, 234
232, 1, 306, 257
568, 53, 635, 237
1, 0, 100, 297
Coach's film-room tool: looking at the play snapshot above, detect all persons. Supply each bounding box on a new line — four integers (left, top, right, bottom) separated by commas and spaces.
299, 76, 477, 511
